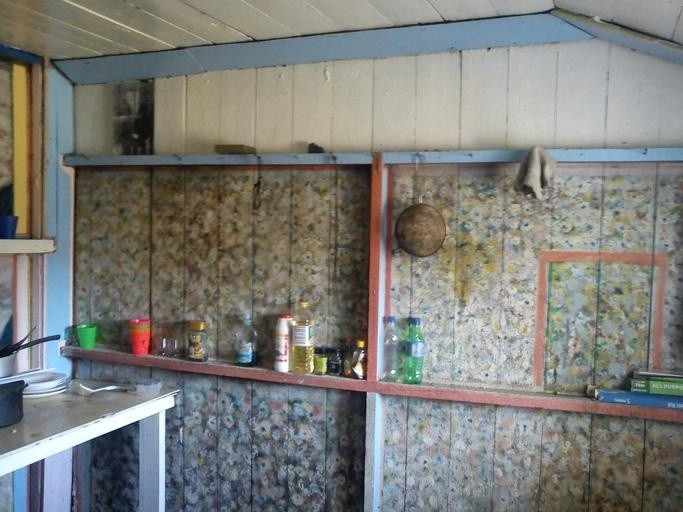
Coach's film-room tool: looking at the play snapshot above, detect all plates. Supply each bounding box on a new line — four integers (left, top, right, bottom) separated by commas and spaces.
12, 371, 69, 398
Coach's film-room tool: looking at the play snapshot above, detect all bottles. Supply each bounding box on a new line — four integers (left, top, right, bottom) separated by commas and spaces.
188, 300, 425, 385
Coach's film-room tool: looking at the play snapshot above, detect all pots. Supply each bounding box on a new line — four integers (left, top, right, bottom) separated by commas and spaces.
1, 383, 28, 429
0, 335, 62, 378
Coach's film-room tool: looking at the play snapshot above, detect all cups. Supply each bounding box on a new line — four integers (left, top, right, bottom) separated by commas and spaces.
76, 318, 186, 359
0, 216, 18, 240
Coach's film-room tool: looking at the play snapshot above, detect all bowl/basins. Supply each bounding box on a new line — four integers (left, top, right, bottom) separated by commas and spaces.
134, 377, 161, 396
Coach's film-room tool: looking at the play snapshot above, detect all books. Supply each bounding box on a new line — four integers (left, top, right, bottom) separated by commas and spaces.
585, 366, 683, 409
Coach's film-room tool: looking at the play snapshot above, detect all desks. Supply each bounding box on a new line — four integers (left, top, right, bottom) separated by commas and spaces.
1, 378, 180, 509
